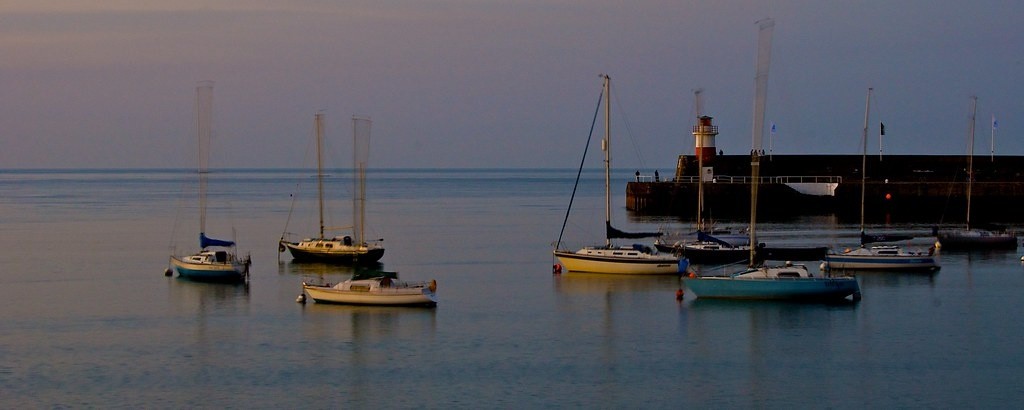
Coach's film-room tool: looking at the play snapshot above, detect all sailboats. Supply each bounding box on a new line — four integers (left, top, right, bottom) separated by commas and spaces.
819, 86, 942, 272
934, 92, 1019, 255
677, 23, 860, 305
280, 112, 385, 265
551, 73, 688, 278
656, 88, 765, 267
166, 81, 251, 285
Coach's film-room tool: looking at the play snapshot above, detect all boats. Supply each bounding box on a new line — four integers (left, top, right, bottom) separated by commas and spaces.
298, 270, 438, 308
753, 245, 829, 262
